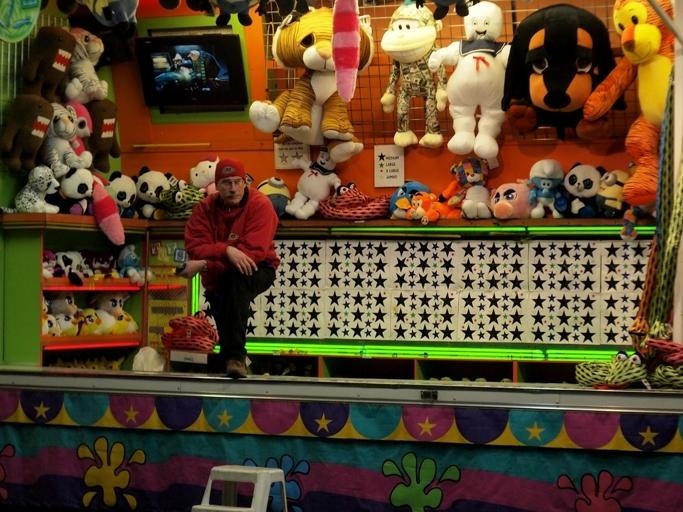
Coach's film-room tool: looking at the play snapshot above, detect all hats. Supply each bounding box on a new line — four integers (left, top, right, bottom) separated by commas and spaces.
213, 157, 247, 185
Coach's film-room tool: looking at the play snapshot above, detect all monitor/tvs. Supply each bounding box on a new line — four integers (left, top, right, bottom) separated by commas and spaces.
133, 34, 249, 114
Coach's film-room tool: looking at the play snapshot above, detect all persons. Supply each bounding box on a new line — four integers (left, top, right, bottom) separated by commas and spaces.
171, 158, 279, 378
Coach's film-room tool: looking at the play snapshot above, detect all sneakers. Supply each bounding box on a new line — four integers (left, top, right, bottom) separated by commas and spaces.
224, 359, 247, 379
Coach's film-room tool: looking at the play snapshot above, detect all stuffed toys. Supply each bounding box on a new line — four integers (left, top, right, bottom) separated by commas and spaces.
1, 25, 215, 372
256, 2, 673, 240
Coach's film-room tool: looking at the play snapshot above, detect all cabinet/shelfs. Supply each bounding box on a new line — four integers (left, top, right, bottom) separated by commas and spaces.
0, 212, 192, 377
